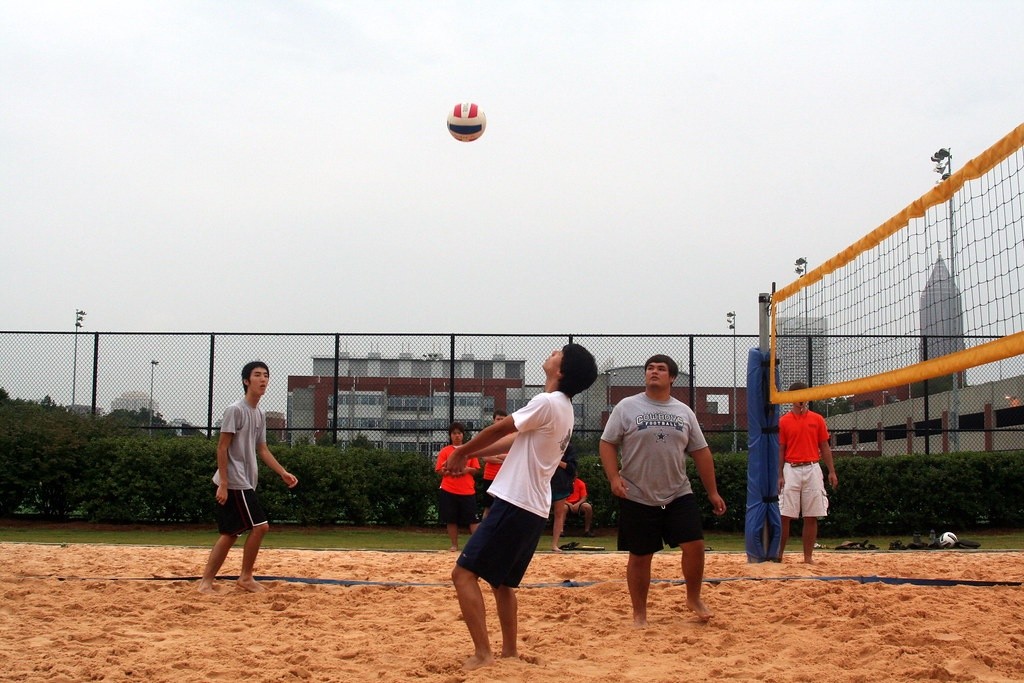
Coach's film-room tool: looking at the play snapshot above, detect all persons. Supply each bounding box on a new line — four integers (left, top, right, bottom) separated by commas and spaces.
197, 361, 299, 594
433, 420, 481, 553
550, 441, 580, 554
559, 475, 595, 538
601, 354, 728, 630
482, 410, 511, 520
775, 381, 839, 565
439, 343, 600, 670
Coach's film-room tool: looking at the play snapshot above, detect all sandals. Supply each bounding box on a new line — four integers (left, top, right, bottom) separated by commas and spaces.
856, 540, 880, 550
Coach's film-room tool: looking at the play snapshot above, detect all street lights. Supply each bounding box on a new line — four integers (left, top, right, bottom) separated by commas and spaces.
725, 310, 739, 452
149, 359, 160, 427
930, 146, 963, 453
71, 307, 87, 404
794, 258, 816, 389
601, 370, 617, 413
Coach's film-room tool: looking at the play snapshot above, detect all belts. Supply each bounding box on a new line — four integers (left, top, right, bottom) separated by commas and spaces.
786, 461, 818, 467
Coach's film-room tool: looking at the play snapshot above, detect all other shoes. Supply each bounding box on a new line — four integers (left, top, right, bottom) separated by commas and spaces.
889, 541, 909, 550
584, 531, 595, 537
560, 531, 565, 537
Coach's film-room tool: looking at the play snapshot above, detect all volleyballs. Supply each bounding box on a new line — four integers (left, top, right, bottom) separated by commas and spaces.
940, 531, 957, 548
447, 102, 487, 142
